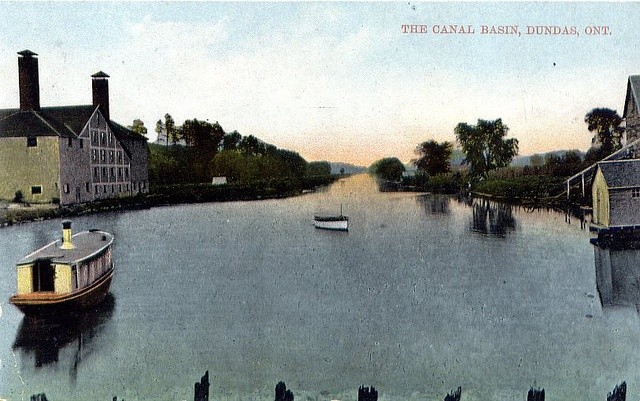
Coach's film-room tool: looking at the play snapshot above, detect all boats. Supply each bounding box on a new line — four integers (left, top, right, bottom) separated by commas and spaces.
9, 220, 115, 318
314, 203, 349, 230
